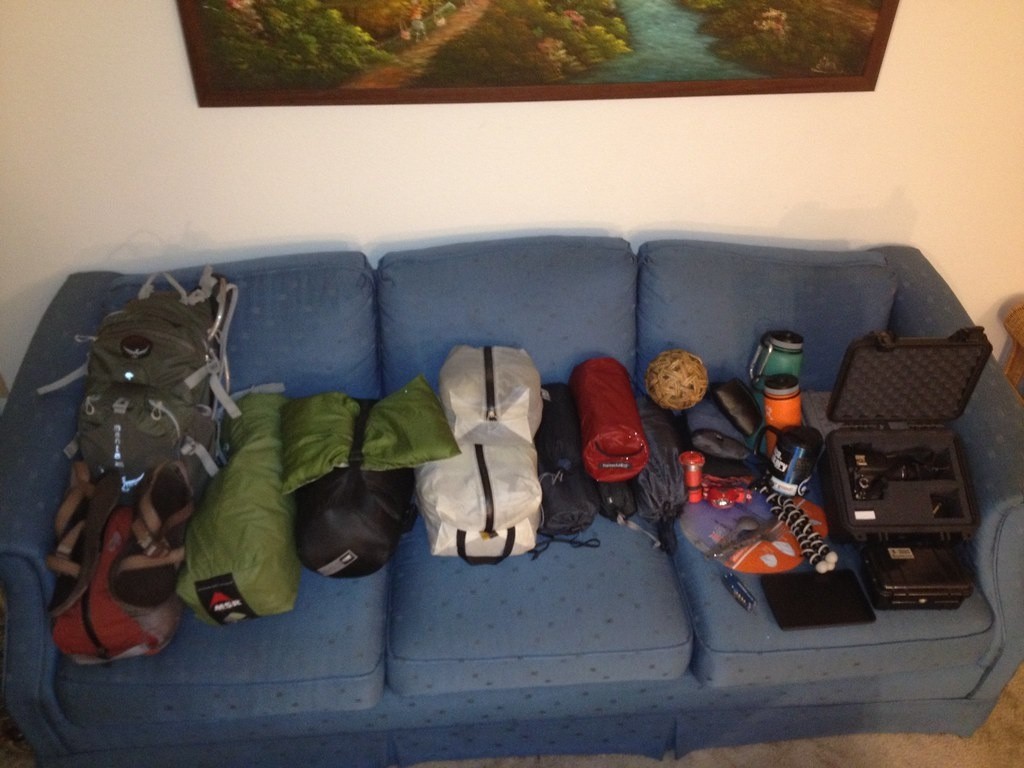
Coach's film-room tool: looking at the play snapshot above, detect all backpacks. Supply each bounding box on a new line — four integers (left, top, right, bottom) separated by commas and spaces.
37, 262, 242, 502
178, 381, 300, 627
296, 372, 420, 578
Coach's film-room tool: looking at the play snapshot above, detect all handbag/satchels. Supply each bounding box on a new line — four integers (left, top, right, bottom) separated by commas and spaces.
529, 356, 688, 561
45, 455, 199, 666
416, 341, 544, 567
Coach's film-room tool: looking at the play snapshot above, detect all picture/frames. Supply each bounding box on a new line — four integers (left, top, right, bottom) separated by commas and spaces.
176, 0, 899, 108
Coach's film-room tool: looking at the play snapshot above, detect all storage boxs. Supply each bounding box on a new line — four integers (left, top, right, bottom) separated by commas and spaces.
861, 546, 974, 610
817, 324, 993, 544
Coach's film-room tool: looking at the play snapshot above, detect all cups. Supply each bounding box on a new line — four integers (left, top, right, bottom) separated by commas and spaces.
772, 436, 812, 494
763, 374, 802, 461
754, 330, 803, 413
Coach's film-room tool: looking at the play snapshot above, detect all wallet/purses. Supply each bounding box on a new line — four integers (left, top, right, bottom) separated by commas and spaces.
714, 377, 763, 438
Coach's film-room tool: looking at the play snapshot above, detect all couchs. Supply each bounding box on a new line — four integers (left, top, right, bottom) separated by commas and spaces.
0, 233, 1024, 768
